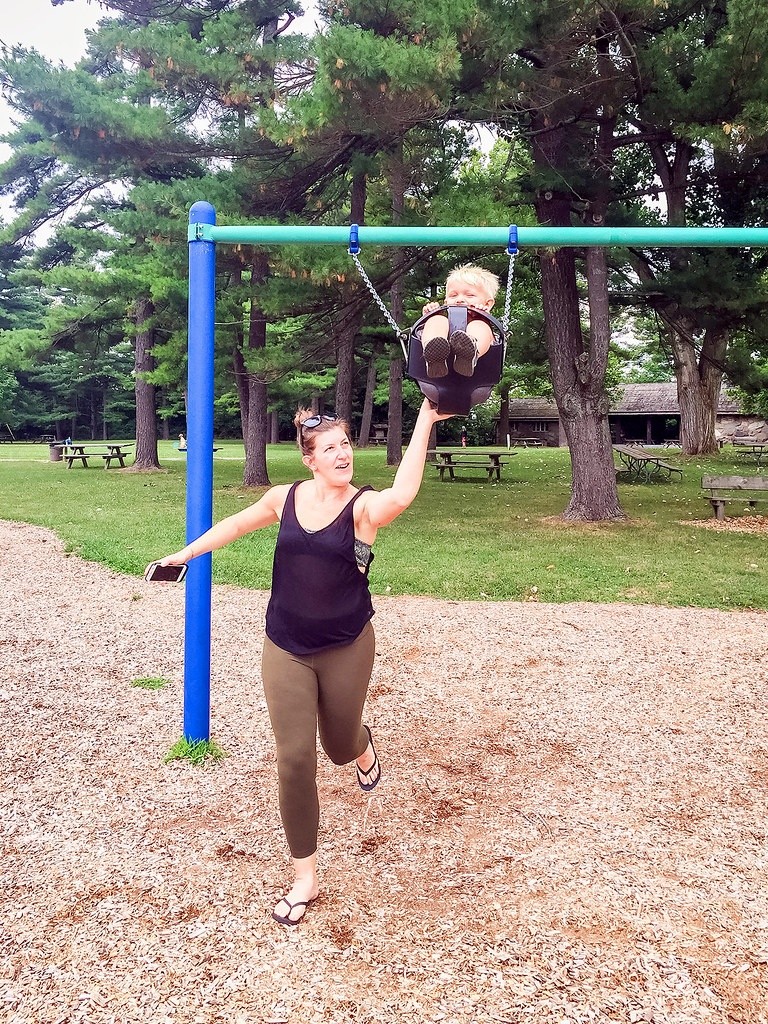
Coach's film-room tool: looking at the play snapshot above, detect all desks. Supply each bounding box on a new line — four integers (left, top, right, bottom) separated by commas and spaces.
55, 443, 134, 467
427, 450, 518, 478
625, 439, 645, 448
512, 438, 539, 449
735, 443, 768, 460
0, 434, 57, 444
369, 437, 387, 444
663, 439, 681, 449
613, 446, 660, 482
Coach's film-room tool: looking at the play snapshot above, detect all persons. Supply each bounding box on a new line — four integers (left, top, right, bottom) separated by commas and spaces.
415, 262, 499, 407
461, 426, 467, 449
145, 395, 456, 923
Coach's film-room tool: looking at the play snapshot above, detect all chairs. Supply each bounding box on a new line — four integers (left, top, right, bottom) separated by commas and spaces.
651, 460, 683, 480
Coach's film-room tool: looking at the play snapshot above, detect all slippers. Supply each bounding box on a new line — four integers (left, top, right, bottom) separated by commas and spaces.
272, 894, 319, 925
356, 725, 381, 791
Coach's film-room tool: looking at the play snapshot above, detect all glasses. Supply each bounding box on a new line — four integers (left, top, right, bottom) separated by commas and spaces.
300, 412, 340, 456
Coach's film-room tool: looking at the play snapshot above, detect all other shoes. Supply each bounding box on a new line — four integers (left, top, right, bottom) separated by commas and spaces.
420, 336, 450, 377
449, 330, 480, 377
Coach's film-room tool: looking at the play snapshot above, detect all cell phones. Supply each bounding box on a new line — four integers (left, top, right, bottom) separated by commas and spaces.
145, 564, 188, 582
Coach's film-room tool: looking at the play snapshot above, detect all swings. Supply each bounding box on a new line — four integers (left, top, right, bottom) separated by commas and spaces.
346, 224, 518, 417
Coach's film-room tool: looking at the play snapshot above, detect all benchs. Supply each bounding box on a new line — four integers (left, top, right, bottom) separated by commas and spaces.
59, 455, 113, 469
524, 442, 542, 449
614, 466, 631, 476
701, 475, 768, 521
735, 450, 768, 457
756, 453, 768, 466
430, 463, 500, 480
445, 460, 510, 475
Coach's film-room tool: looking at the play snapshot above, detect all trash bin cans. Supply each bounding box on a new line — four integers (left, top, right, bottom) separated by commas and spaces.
49, 444, 67, 461
